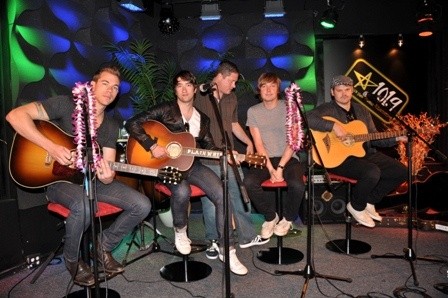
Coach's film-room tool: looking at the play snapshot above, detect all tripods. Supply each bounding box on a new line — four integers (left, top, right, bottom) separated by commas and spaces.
121, 179, 183, 266
369, 98, 447, 285
274, 97, 351, 298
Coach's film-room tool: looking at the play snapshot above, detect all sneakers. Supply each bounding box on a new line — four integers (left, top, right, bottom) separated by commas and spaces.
206, 240, 218, 259
239, 235, 270, 248
366, 203, 383, 222
220, 248, 248, 275
262, 213, 279, 238
174, 227, 192, 255
346, 202, 375, 228
274, 217, 294, 236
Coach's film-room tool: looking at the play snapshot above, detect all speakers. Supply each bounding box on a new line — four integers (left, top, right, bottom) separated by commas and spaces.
302, 171, 348, 224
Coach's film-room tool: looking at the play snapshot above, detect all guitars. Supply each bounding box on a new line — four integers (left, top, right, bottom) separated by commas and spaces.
308, 116, 424, 170
124, 120, 268, 171
8, 118, 183, 190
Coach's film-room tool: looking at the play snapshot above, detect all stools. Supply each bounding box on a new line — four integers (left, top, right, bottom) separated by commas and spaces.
31, 172, 372, 298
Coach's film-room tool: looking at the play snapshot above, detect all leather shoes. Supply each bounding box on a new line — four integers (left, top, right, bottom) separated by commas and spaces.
96, 250, 126, 273
65, 258, 95, 286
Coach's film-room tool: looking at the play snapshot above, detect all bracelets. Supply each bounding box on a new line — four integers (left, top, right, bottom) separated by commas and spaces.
150, 144, 158, 151
278, 165, 284, 169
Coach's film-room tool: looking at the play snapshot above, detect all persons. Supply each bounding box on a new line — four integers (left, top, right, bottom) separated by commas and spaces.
194, 60, 271, 259
5, 66, 151, 286
246, 72, 305, 239
305, 75, 409, 228
125, 70, 248, 276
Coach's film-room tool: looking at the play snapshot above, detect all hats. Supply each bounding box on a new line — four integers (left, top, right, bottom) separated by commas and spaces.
331, 75, 353, 87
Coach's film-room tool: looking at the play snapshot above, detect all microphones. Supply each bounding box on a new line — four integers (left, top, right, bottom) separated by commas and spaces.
199, 82, 216, 93
363, 91, 382, 98
